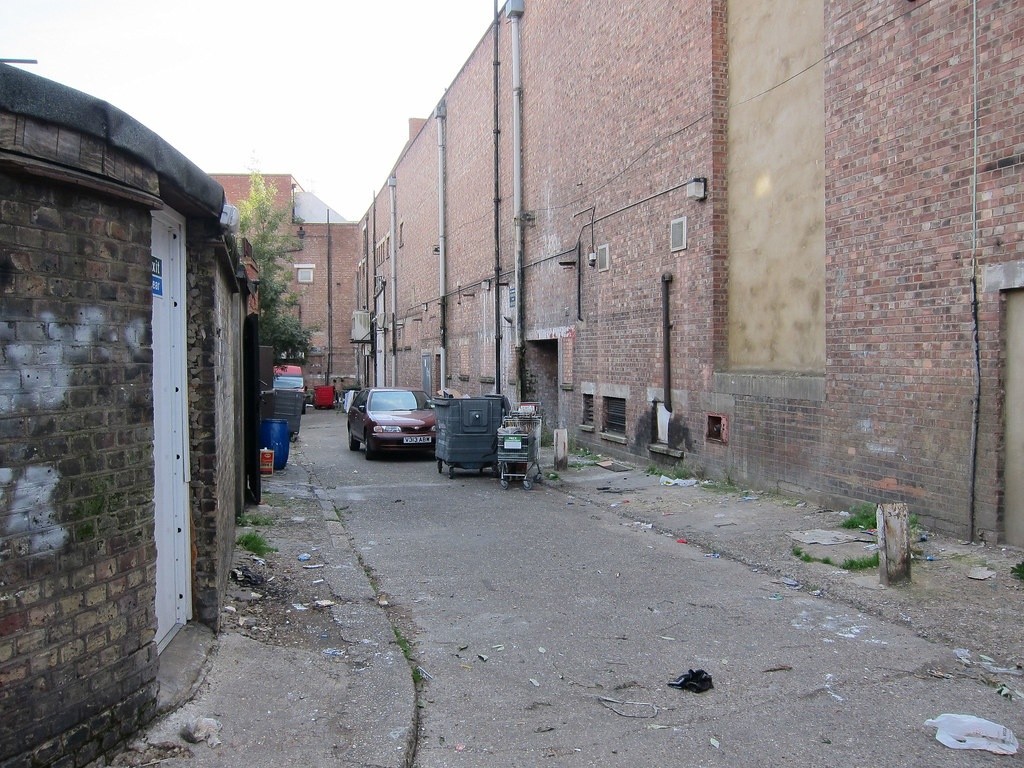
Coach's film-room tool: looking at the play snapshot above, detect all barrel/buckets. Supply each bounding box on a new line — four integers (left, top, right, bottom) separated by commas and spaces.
259, 418, 289, 471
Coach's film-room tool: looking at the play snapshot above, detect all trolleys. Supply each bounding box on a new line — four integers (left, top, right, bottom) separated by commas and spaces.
497, 402, 542, 490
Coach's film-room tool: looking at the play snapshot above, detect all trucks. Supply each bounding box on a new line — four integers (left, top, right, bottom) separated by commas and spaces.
273, 364, 307, 415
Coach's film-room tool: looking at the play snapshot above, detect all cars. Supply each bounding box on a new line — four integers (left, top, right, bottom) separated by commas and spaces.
347, 386, 438, 460
